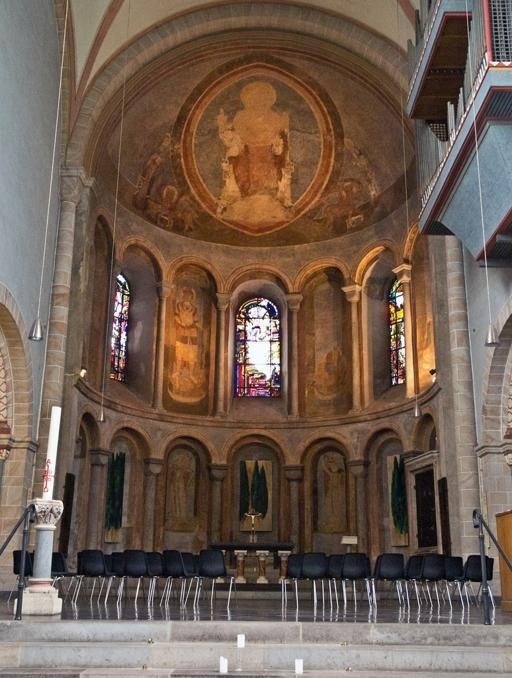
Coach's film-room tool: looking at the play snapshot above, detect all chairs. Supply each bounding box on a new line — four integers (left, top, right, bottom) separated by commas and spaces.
6, 549, 496, 624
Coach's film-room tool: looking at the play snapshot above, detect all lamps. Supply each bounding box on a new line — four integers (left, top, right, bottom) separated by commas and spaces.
97, 1, 131, 423
396, 0, 422, 418
28, 0, 69, 341
465, 0, 501, 345
